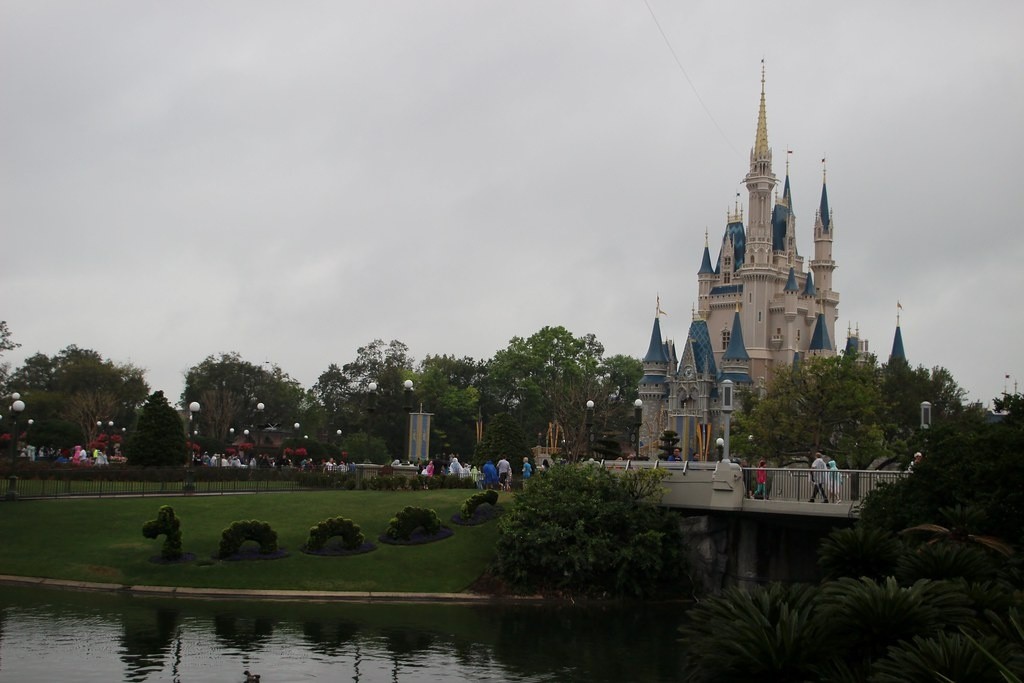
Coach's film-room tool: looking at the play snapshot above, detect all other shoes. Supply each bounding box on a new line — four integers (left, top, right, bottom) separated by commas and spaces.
750, 496, 755, 500
821, 499, 829, 503
764, 497, 768, 501
836, 500, 841, 504
808, 499, 814, 502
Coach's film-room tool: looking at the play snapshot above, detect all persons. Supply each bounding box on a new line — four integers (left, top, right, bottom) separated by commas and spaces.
808, 452, 829, 503
522, 457, 532, 479
693, 452, 700, 461
250, 453, 272, 467
608, 455, 623, 470
301, 458, 355, 472
823, 456, 843, 504
496, 455, 512, 491
410, 457, 484, 490
668, 448, 682, 461
908, 452, 922, 471
73, 445, 109, 465
750, 461, 766, 500
204, 452, 245, 466
483, 458, 499, 489
26, 443, 61, 461
741, 459, 752, 496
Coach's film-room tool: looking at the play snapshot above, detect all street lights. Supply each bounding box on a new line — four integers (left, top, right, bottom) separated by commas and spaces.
293, 422, 308, 451
363, 382, 377, 464
182, 401, 201, 493
230, 403, 264, 450
585, 400, 594, 461
721, 380, 733, 460
402, 379, 412, 466
0, 393, 34, 501
336, 430, 342, 466
635, 399, 643, 461
717, 438, 724, 462
97, 421, 126, 462
